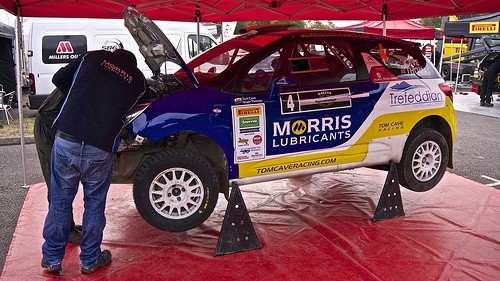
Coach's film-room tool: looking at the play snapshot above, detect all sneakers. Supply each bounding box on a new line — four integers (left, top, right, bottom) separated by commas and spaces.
41, 259, 63, 272
80, 250, 112, 274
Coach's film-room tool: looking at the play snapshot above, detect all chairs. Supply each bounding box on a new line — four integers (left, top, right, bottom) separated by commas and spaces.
0, 91, 18, 125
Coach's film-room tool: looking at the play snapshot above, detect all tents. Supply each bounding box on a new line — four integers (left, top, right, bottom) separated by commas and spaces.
338, 18, 436, 76
439, 13, 500, 96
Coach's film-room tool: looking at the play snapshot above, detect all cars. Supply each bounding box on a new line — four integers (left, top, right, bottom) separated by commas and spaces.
107, 5, 457, 232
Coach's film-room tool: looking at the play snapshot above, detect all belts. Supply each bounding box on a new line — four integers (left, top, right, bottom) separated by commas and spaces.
58, 131, 82, 143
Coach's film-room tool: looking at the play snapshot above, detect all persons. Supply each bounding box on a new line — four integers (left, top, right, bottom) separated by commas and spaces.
33, 37, 124, 245
479, 59, 500, 107
41, 48, 154, 275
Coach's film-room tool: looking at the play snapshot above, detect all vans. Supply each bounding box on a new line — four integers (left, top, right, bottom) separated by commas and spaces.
25, 17, 232, 110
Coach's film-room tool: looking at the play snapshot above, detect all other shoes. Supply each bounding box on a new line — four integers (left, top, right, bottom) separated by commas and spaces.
68, 225, 83, 243
485, 102, 493, 107
479, 101, 484, 106
